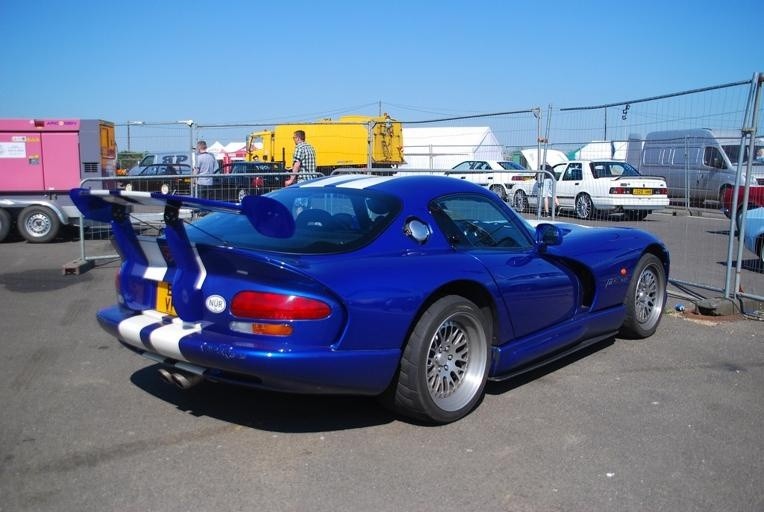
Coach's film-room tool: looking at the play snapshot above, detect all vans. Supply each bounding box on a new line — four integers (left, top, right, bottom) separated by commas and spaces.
125, 151, 193, 179
638, 126, 764, 205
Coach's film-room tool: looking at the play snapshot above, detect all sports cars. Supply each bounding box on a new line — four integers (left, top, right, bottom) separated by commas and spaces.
71, 171, 673, 426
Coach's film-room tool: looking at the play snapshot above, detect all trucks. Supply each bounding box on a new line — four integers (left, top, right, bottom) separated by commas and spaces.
0, 115, 119, 243
242, 112, 405, 182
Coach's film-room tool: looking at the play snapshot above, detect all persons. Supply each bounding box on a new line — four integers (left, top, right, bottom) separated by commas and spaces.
534, 162, 562, 218
285, 131, 317, 185
192, 140, 220, 200
166, 164, 177, 192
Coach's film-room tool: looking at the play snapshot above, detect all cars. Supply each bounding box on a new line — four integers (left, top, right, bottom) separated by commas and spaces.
119, 162, 198, 195
441, 159, 537, 203
509, 159, 670, 224
193, 159, 292, 202
718, 184, 763, 231
734, 207, 763, 268
